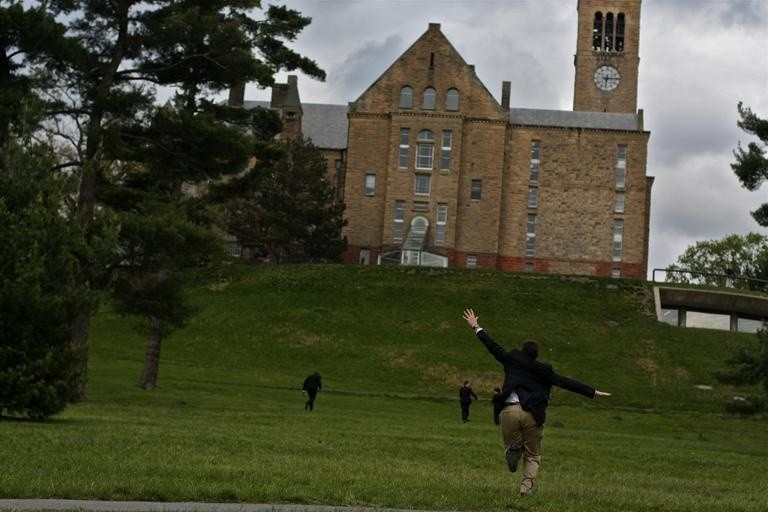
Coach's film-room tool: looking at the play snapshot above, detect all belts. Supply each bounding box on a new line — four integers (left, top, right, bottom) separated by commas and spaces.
503, 400, 521, 407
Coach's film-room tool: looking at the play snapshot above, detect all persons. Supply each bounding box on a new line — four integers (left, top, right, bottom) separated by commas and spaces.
300, 370, 323, 411
459, 379, 479, 423
461, 302, 613, 496
487, 387, 504, 426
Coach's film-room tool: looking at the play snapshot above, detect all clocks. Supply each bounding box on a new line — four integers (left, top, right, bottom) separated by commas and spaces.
593, 65, 620, 92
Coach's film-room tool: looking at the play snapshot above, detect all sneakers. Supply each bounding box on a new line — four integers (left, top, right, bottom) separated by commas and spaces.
518, 491, 533, 497
509, 431, 522, 453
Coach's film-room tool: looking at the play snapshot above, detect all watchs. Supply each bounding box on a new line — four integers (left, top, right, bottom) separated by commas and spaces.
474, 324, 479, 330
